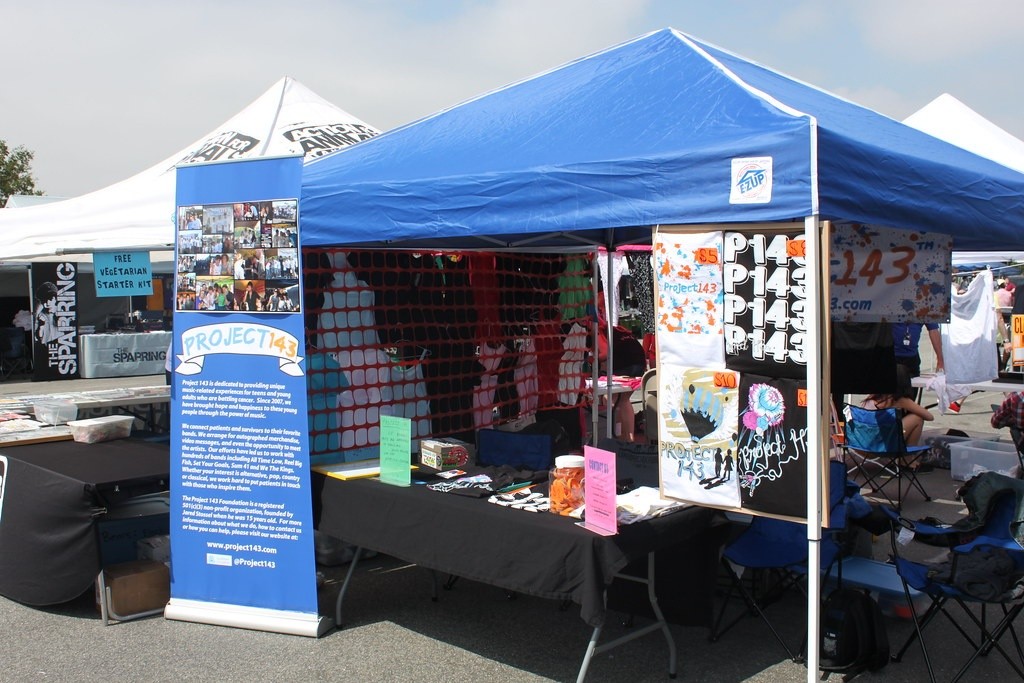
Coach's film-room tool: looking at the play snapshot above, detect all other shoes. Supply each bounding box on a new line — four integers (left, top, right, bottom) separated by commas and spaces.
889, 463, 934, 474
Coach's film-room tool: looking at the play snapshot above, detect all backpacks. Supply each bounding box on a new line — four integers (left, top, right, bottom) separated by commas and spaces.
803, 588, 890, 682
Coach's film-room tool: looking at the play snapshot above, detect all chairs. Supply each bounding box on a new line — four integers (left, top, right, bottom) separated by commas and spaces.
879, 472, 1024, 683
837, 401, 931, 513
707, 458, 858, 664
0, 327, 31, 382
474, 427, 553, 472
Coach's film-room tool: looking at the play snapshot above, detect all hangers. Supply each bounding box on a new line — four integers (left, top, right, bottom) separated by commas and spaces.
304, 327, 320, 352
473, 318, 512, 355
304, 289, 592, 366
383, 322, 432, 361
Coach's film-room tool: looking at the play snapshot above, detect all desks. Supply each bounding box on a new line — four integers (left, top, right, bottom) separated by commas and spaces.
910, 376, 1024, 406
78, 330, 174, 379
311, 451, 718, 683
0, 385, 170, 606
583, 374, 633, 452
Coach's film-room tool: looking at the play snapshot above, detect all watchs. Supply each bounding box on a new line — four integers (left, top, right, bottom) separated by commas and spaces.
1003, 339, 1010, 343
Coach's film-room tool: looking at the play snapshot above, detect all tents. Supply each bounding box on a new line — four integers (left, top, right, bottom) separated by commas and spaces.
0, 74, 384, 276
301, 25, 1024, 683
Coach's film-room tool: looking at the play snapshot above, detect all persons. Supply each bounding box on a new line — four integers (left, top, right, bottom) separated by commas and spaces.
862, 364, 934, 472
177, 200, 299, 311
999, 284, 1024, 371
951, 273, 1016, 306
890, 323, 944, 417
991, 391, 1024, 457
994, 291, 1013, 370
569, 308, 647, 443
34, 282, 65, 344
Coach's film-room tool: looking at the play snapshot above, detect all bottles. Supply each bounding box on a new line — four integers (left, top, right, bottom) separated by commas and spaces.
548, 455, 585, 517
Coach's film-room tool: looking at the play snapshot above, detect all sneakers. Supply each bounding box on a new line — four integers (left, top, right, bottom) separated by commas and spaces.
937, 400, 961, 413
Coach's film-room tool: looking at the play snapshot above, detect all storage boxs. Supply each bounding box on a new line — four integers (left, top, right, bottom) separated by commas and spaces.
314, 527, 378, 567
136, 533, 170, 565
66, 415, 135, 444
95, 558, 170, 622
95, 491, 170, 565
33, 399, 78, 425
822, 555, 943, 623
948, 439, 1024, 483
914, 425, 1002, 473
131, 430, 170, 446
